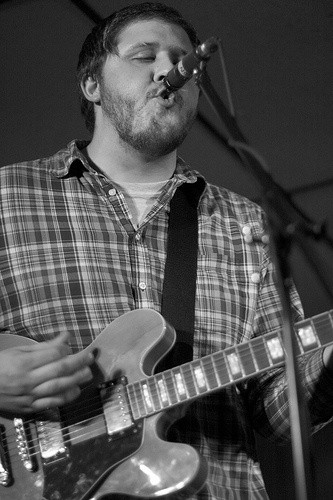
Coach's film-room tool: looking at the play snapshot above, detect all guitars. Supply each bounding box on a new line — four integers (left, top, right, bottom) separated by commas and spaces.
0, 308, 333, 500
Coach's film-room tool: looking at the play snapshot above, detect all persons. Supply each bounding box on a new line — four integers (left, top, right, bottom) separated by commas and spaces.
1, 0, 332, 500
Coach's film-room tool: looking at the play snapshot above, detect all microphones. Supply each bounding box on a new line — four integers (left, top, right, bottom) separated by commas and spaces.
161, 36, 218, 93
